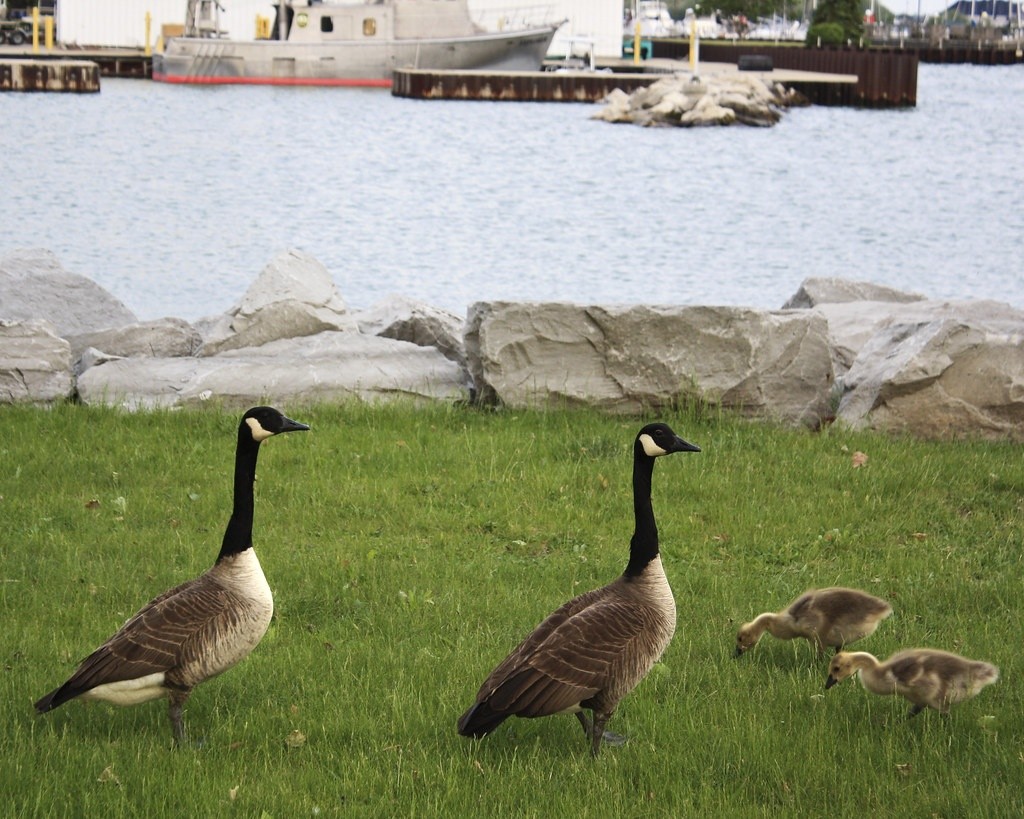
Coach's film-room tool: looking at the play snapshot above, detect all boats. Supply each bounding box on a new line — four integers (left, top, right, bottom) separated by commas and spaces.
148, 0, 568, 87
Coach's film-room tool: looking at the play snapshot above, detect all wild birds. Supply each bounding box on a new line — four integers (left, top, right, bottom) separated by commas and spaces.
457, 423, 998, 759
32, 406, 312, 747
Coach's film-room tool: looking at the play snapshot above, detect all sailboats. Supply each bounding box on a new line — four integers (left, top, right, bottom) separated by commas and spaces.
620, 0, 1024, 73
